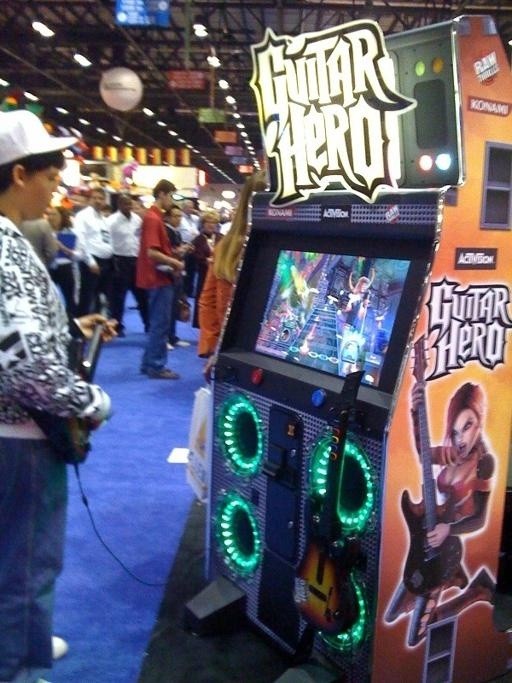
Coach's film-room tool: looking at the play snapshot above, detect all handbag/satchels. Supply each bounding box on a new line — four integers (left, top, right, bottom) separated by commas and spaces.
175, 301, 190, 321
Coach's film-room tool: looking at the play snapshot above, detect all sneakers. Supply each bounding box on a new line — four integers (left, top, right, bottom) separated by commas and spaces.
141, 365, 178, 379
166, 340, 190, 351
47, 636, 68, 660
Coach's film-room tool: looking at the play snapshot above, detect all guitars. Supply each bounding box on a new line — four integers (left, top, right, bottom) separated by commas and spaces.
291, 370, 365, 636
156, 243, 196, 277
402, 333, 462, 595
27, 319, 119, 464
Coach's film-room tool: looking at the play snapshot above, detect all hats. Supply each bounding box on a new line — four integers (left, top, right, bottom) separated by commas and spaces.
0, 109, 79, 165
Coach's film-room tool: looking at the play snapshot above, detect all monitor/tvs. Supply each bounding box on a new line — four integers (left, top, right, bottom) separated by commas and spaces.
234, 229, 434, 395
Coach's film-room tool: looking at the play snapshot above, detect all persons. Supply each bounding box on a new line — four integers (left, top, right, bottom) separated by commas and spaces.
196, 168, 271, 385
18, 175, 231, 380
0, 106, 121, 683
